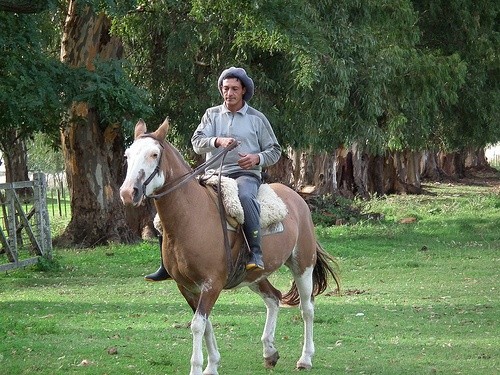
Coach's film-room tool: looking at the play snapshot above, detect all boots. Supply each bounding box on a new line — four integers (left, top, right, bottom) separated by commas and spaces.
245, 223, 265, 272
144, 235, 173, 282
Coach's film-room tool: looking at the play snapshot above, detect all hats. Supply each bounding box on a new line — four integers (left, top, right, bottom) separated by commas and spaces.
218, 66, 254, 104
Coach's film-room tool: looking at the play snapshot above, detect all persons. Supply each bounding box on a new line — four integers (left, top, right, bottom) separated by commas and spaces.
143, 67, 282, 281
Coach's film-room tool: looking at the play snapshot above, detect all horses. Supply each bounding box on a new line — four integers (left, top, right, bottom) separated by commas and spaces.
119, 116, 342, 375
